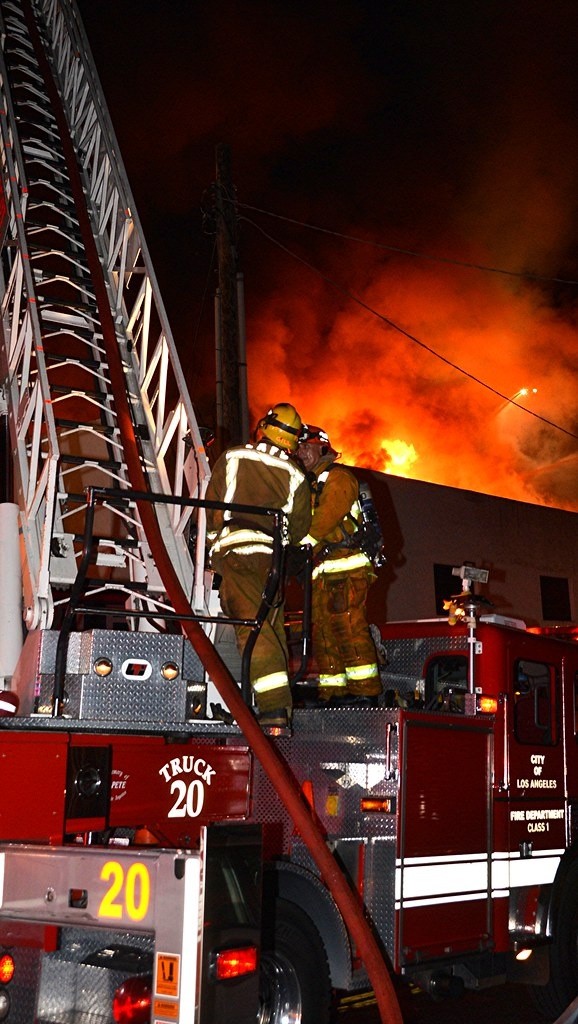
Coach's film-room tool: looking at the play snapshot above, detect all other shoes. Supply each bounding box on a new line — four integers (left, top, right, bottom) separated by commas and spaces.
305, 696, 341, 708
257, 709, 288, 727
340, 692, 374, 709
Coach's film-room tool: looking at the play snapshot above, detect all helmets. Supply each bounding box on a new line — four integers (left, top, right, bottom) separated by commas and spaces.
299, 425, 330, 449
263, 403, 301, 450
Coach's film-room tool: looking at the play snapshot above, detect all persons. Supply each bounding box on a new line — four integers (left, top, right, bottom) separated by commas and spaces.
207, 403, 312, 727
296, 425, 383, 708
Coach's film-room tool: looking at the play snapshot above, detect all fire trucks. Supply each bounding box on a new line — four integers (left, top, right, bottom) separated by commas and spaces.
0, 0, 578, 1024
